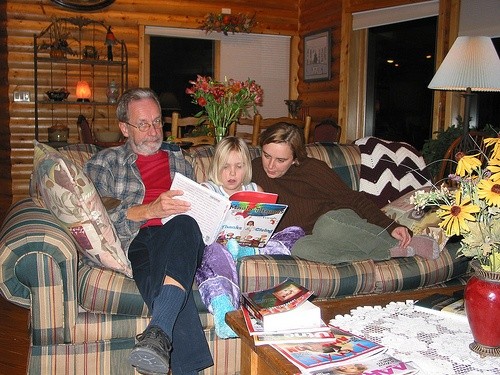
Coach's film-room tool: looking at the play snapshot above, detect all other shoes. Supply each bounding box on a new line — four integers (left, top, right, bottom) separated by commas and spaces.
128, 324, 172, 375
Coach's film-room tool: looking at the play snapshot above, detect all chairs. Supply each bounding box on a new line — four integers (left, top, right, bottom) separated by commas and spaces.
171, 112, 340, 146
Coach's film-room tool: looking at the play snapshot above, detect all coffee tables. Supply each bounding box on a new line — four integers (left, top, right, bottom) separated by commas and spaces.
225, 285, 500, 374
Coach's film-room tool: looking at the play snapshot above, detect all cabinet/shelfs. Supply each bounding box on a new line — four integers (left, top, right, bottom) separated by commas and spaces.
34, 16, 128, 148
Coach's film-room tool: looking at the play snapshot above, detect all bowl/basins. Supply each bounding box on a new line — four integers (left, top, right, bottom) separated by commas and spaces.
47, 125, 70, 142
46, 91, 70, 101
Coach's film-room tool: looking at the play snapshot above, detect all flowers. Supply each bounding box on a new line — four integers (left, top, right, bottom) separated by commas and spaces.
200, 11, 259, 39
376, 123, 500, 273
185, 74, 263, 142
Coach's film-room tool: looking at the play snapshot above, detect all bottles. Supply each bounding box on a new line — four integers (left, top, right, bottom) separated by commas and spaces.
106, 79, 119, 104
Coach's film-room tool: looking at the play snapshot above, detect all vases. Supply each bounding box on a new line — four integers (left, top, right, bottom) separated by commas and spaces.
214, 126, 228, 145
465, 260, 500, 358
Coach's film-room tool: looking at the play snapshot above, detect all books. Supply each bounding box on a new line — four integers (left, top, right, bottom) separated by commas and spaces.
240, 277, 419, 375
160, 171, 288, 248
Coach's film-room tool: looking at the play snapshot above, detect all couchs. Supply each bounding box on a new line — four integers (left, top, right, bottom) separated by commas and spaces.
0, 143, 471, 375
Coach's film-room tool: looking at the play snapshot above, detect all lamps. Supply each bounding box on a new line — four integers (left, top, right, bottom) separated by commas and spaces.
427, 36, 500, 153
76, 80, 91, 103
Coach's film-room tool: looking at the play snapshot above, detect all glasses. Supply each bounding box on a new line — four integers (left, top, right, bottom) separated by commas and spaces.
122, 120, 165, 132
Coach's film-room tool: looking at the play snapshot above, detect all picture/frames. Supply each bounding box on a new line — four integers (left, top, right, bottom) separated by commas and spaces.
303, 28, 331, 82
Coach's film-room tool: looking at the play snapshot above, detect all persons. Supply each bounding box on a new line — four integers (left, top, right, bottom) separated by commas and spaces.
250, 122, 439, 264
195, 136, 305, 339
84, 87, 214, 375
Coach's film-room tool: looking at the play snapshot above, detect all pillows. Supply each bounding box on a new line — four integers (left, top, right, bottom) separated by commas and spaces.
380, 186, 460, 253
32, 140, 133, 280
